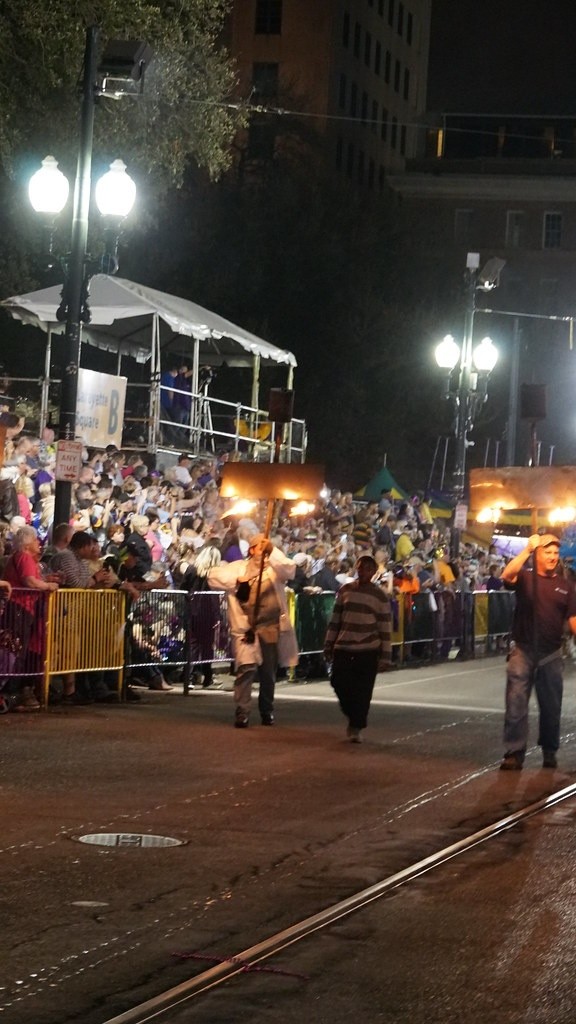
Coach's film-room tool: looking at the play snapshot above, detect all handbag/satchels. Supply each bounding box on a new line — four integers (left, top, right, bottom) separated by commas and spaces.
239, 578, 281, 626
429, 592, 438, 612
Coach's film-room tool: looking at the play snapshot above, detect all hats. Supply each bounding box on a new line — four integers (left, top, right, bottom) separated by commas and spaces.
293, 553, 312, 567
408, 556, 426, 566
540, 534, 562, 547
249, 533, 271, 547
178, 453, 192, 463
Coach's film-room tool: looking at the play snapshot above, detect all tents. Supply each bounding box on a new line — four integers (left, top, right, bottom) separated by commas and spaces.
7, 276, 295, 451
351, 469, 452, 519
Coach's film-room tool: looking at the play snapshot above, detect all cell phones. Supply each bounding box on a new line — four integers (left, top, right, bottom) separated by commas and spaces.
102, 561, 109, 572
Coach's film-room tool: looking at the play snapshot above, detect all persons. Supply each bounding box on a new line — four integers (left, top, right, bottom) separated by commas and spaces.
160, 365, 210, 434
208, 534, 296, 727
0, 412, 518, 712
323, 556, 392, 743
500, 532, 576, 770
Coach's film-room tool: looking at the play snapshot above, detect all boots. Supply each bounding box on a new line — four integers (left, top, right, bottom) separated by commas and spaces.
24, 686, 40, 708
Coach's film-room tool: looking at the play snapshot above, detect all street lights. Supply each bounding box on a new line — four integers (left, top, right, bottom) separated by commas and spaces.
23, 149, 140, 551
434, 334, 502, 573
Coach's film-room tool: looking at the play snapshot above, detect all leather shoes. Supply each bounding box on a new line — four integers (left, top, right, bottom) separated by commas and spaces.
234, 713, 249, 727
260, 711, 275, 725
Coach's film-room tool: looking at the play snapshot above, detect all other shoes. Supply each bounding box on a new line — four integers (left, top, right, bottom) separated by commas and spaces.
120, 687, 141, 701
213, 678, 223, 684
500, 752, 524, 770
201, 684, 223, 689
347, 719, 352, 737
542, 750, 557, 767
350, 731, 362, 742
